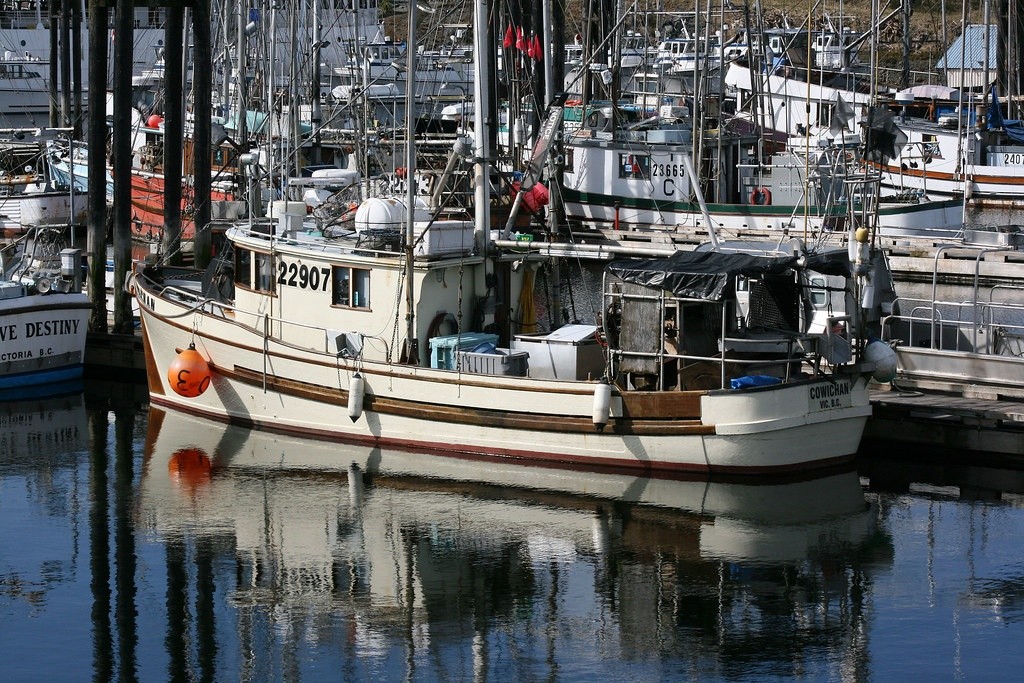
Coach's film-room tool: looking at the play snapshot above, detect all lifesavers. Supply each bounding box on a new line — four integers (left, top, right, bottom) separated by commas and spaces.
750, 187, 771, 205
1, 175, 39, 184
566, 99, 582, 106
427, 311, 458, 358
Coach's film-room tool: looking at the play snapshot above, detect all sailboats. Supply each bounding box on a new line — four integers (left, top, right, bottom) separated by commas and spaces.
0, 0, 1024, 480
